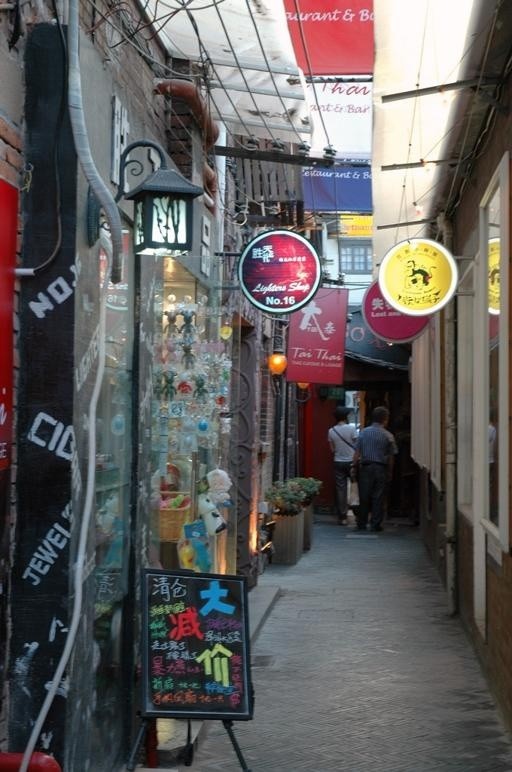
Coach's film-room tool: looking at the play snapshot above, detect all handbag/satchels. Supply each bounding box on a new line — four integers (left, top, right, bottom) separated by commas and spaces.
347, 477, 360, 506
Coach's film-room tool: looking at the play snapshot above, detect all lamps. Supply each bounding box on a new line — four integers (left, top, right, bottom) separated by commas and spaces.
267, 335, 288, 398
87, 139, 204, 259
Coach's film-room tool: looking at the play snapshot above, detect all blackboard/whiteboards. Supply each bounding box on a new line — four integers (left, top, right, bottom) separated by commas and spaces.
141, 569, 250, 720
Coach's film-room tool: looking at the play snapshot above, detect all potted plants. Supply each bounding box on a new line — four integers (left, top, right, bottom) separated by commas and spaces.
265, 481, 307, 565
288, 477, 323, 552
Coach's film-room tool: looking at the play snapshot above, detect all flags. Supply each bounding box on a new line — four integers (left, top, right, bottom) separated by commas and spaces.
282, 286, 351, 385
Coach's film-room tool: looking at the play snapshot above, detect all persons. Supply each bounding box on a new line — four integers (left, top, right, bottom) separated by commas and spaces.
350, 406, 397, 533
488, 407, 498, 485
327, 406, 364, 525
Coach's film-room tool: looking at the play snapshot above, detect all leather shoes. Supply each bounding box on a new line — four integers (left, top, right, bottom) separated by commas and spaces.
336, 516, 384, 532
